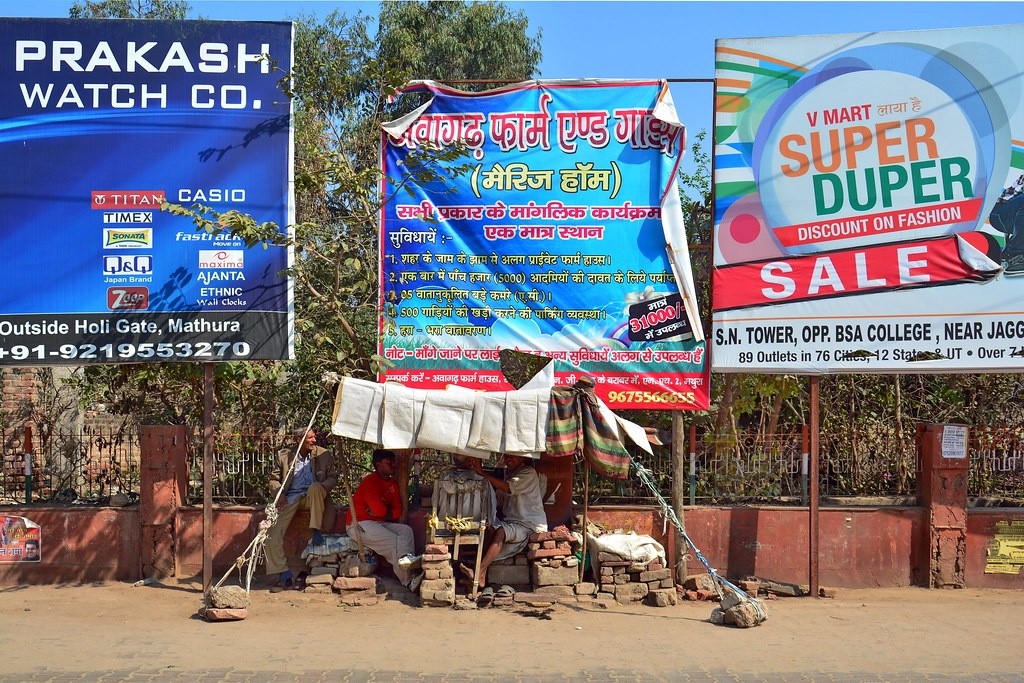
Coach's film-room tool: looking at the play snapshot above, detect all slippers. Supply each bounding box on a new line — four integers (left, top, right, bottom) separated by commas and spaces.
481, 586, 493, 598
496, 584, 515, 597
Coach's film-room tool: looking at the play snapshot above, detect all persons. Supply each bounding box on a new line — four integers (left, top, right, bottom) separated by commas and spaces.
345, 447, 424, 591
262, 426, 338, 589
431, 451, 497, 531
459, 453, 548, 592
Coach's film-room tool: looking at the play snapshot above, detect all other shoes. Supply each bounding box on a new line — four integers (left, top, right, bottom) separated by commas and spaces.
294, 571, 308, 586
276, 578, 292, 587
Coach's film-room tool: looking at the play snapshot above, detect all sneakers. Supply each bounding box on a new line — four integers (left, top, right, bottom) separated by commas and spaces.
398, 554, 422, 571
408, 571, 425, 592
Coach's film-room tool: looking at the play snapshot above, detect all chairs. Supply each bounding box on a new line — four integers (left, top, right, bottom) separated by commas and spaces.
427, 478, 489, 600
